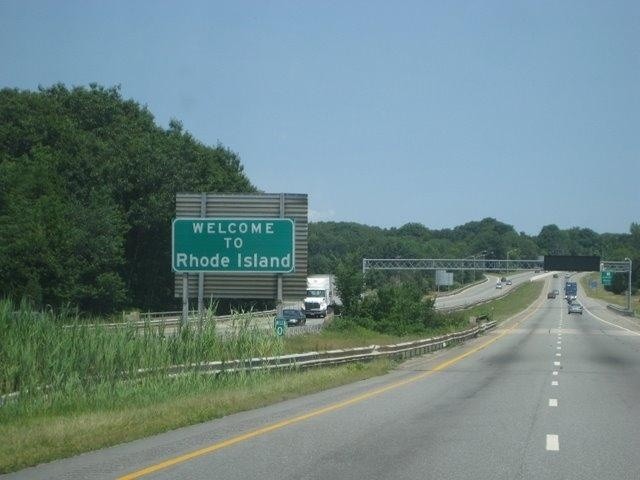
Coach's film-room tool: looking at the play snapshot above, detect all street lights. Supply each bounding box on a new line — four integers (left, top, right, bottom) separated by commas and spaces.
507, 248, 519, 277
625, 255, 634, 313
461, 249, 495, 287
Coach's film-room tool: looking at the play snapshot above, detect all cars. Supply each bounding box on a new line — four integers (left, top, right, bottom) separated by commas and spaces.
497, 277, 513, 289
547, 272, 585, 316
273, 308, 308, 327
534, 267, 550, 274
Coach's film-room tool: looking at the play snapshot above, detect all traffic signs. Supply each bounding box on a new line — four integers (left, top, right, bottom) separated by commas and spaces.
601, 268, 615, 286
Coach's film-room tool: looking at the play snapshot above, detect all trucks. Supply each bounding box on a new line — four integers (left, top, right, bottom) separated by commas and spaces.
301, 273, 339, 319
